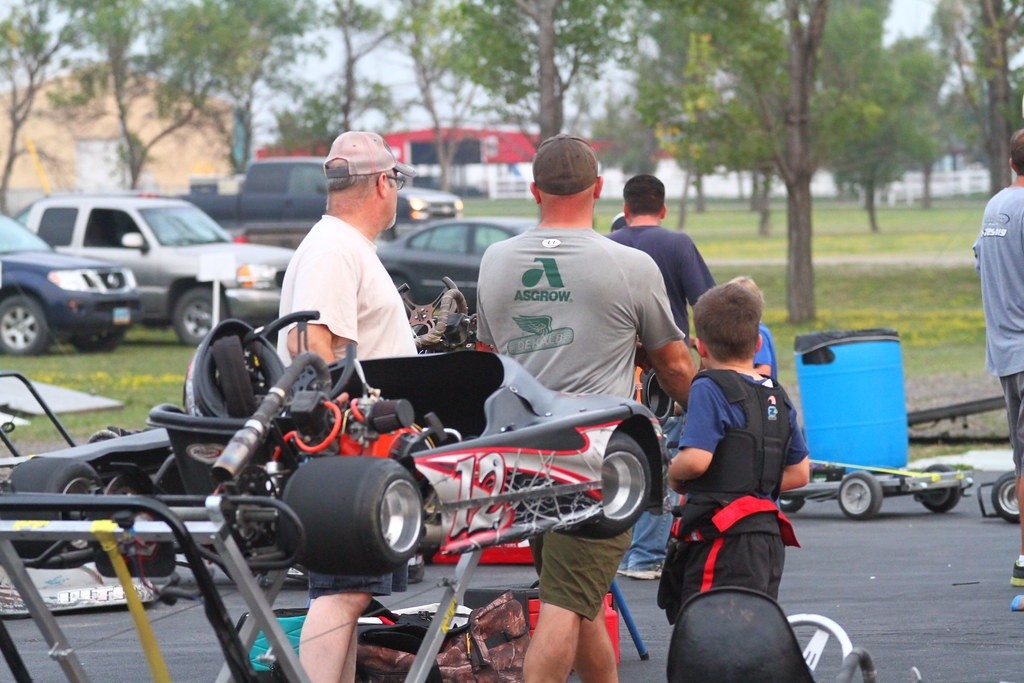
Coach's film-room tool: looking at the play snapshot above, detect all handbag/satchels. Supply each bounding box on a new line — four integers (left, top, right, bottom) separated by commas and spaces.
355, 589, 532, 683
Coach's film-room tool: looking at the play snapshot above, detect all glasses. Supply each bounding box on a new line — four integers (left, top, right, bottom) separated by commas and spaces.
376, 175, 406, 190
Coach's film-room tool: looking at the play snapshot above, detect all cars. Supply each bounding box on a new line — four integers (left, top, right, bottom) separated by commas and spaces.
1, 210, 144, 356
372, 212, 541, 320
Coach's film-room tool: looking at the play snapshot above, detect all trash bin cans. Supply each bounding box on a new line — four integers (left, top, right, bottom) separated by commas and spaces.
793, 328, 909, 474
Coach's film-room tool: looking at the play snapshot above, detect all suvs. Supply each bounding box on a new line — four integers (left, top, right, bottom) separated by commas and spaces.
14, 190, 297, 351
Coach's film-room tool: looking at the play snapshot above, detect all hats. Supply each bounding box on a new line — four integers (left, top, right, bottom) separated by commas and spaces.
532, 134, 598, 195
323, 131, 419, 177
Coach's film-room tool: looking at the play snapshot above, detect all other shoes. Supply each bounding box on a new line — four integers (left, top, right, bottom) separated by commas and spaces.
1010, 559, 1024, 586
617, 560, 665, 579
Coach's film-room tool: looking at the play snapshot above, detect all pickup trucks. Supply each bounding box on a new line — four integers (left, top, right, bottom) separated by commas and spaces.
179, 155, 462, 245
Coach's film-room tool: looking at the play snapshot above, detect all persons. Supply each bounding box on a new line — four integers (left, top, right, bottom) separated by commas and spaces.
728, 276, 777, 382
604, 174, 719, 581
471, 131, 696, 683
657, 283, 808, 625
973, 129, 1023, 586
611, 212, 627, 233
275, 130, 423, 683
